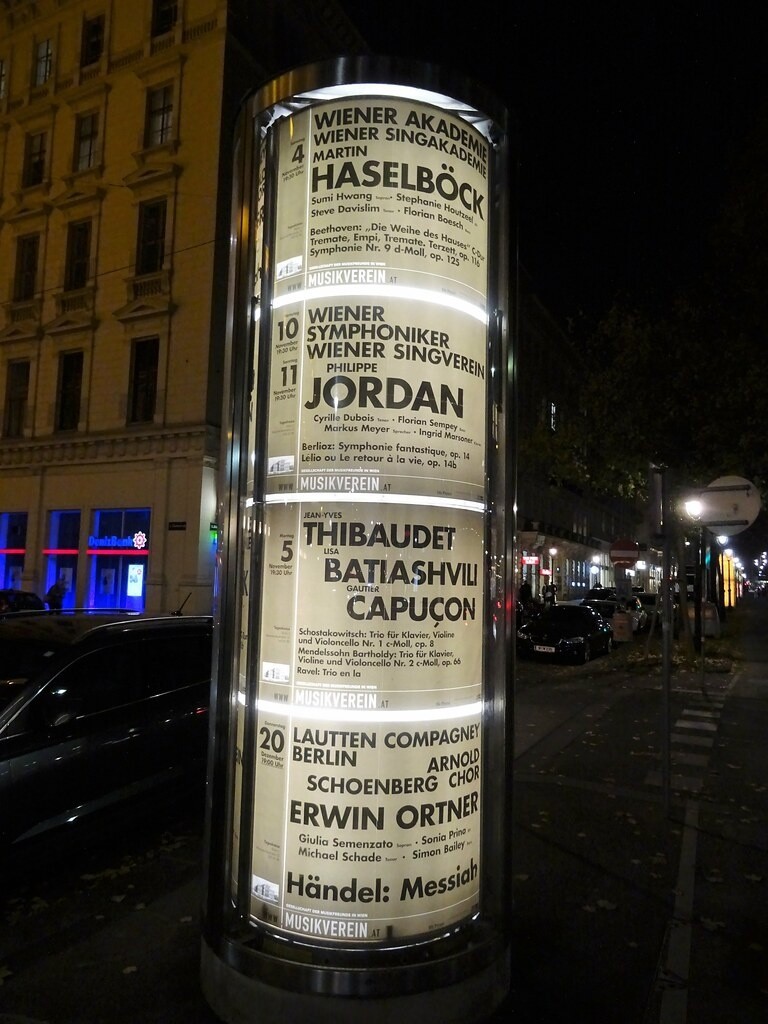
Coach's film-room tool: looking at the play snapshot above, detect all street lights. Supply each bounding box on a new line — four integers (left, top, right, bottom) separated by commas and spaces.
716, 532, 729, 624
548, 547, 558, 585
677, 493, 712, 657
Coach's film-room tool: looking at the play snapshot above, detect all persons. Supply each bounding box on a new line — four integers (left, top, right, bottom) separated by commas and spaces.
754, 589, 762, 600
593, 582, 602, 589
519, 580, 532, 600
46, 580, 66, 615
542, 582, 558, 605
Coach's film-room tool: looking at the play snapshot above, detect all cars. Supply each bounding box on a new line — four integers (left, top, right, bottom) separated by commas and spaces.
579, 584, 696, 645
1, 607, 210, 873
517, 605, 615, 665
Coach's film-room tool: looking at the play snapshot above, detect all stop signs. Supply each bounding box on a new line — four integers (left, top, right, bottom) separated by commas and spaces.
608, 538, 641, 569
